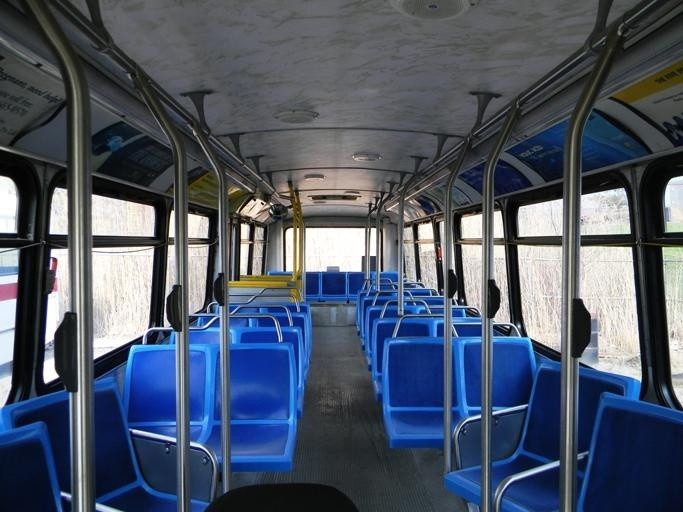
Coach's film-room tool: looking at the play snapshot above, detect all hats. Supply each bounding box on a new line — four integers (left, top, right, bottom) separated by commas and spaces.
0, 247, 64, 371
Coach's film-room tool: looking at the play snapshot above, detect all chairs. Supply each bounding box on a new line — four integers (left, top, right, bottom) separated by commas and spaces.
491, 392, 682, 512
354, 278, 537, 448
303, 269, 410, 305
0, 272, 313, 509
443, 361, 641, 512
